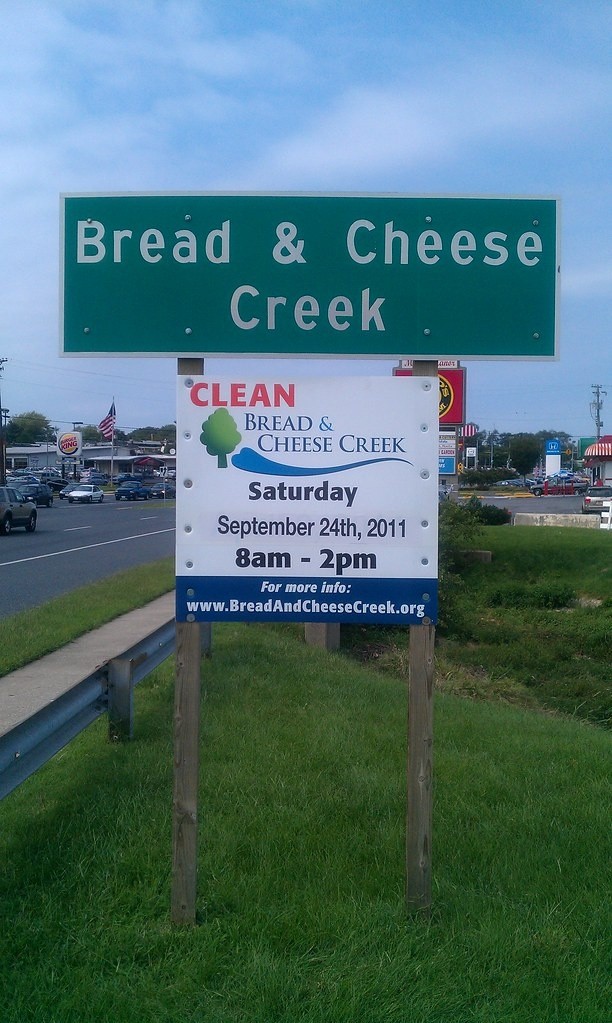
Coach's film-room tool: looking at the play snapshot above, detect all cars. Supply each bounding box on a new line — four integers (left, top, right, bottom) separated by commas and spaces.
47, 479, 70, 492
493, 465, 591, 485
58, 483, 84, 500
166, 470, 176, 479
439, 483, 454, 495
68, 485, 104, 503
79, 468, 106, 478
0, 487, 37, 536
153, 470, 160, 476
5, 465, 78, 484
150, 482, 171, 499
582, 486, 612, 514
17, 484, 53, 509
438, 490, 449, 516
79, 477, 109, 486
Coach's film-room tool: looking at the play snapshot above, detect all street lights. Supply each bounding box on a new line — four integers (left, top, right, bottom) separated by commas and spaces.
72, 420, 83, 479
0, 406, 10, 486
45, 424, 55, 486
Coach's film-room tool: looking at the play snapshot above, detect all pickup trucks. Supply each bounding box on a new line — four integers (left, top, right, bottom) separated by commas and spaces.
529, 476, 588, 496
115, 481, 149, 501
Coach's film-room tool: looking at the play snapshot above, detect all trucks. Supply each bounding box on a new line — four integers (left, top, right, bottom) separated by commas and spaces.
109, 473, 143, 485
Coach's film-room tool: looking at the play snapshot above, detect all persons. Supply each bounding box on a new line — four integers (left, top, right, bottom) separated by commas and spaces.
594, 476, 603, 486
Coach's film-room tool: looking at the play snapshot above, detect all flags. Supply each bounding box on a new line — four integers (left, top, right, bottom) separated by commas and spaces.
98, 404, 115, 439
